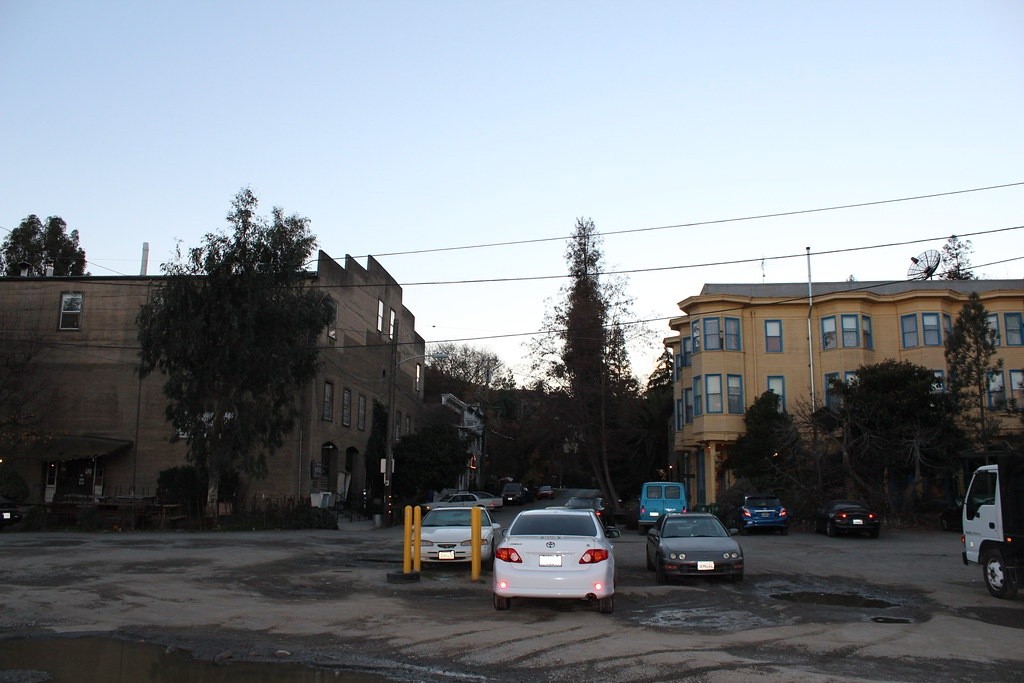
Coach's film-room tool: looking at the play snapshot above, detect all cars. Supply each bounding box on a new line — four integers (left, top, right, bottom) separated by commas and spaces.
812, 500, 880, 539
0, 495, 23, 532
419, 483, 556, 516
492, 510, 621, 614
545, 497, 616, 529
412, 507, 501, 571
940, 497, 988, 532
732, 495, 789, 536
646, 513, 745, 586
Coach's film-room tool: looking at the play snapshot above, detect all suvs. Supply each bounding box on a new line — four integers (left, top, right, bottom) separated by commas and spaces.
637, 482, 690, 536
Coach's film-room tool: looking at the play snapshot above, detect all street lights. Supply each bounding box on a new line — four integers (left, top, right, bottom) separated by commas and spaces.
385, 354, 448, 531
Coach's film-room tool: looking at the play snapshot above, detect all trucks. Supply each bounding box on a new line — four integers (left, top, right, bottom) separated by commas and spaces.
955, 452, 1024, 600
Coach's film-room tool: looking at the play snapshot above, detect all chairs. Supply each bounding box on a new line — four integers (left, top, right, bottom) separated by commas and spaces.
665, 523, 677, 534
